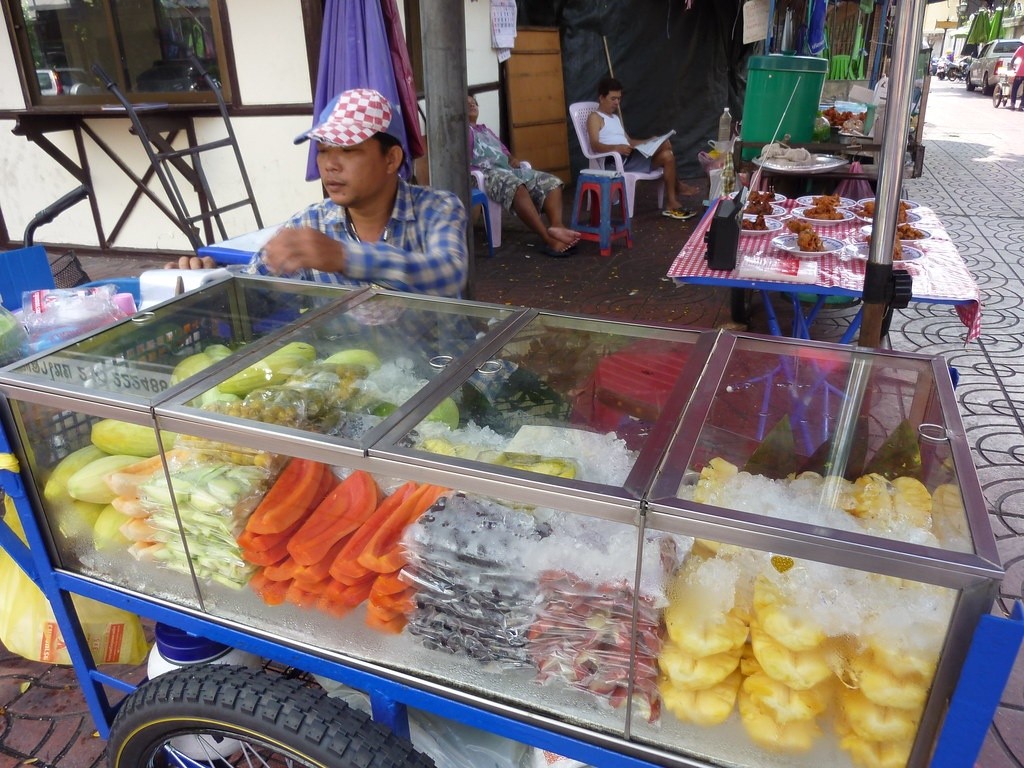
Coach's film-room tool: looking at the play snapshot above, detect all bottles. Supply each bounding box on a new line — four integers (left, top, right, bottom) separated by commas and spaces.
718, 107, 732, 142
146, 621, 263, 761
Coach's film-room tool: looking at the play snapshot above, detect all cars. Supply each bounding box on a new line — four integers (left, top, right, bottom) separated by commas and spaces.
139, 57, 223, 93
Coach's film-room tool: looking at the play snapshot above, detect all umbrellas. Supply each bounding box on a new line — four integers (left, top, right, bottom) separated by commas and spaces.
960, 10, 1003, 57
306, 1, 425, 184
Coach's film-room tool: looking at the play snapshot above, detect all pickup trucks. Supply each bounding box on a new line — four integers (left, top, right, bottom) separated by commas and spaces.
965, 39, 1024, 95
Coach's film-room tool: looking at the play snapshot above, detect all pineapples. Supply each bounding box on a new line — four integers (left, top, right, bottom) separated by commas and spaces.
655, 456, 957, 768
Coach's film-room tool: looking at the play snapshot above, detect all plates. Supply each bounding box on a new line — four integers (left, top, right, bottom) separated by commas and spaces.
860, 225, 933, 242
741, 218, 783, 237
790, 207, 855, 226
855, 212, 923, 224
729, 191, 787, 204
743, 204, 787, 218
795, 196, 856, 209
846, 242, 924, 265
772, 235, 845, 257
857, 198, 921, 211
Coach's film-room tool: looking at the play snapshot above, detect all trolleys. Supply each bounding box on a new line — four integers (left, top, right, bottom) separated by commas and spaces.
992, 64, 1024, 108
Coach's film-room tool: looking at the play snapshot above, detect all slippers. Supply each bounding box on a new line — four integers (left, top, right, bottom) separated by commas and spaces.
677, 186, 700, 196
662, 204, 697, 219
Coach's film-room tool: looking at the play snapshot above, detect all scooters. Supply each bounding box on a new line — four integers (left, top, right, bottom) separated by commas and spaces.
931, 56, 970, 83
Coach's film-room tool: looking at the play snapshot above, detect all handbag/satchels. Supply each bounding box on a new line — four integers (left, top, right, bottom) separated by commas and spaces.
0, 452, 149, 666
832, 161, 875, 203
697, 134, 743, 206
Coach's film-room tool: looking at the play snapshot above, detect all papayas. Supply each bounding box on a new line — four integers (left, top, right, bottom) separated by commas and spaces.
234, 458, 453, 633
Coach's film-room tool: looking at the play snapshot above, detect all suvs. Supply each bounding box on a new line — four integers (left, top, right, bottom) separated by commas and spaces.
35, 67, 93, 100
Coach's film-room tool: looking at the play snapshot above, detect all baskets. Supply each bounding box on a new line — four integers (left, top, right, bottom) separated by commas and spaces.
50, 249, 92, 291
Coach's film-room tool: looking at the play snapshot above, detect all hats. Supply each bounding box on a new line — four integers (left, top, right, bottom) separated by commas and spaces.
1019, 35, 1024, 44
293, 88, 406, 151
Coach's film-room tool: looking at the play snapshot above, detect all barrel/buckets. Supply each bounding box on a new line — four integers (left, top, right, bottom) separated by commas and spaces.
740, 54, 829, 162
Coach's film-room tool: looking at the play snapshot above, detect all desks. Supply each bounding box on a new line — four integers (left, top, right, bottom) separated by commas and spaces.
669, 194, 984, 458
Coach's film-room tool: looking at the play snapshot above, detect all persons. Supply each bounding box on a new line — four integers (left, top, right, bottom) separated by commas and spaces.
946, 51, 952, 77
165, 89, 477, 361
1006, 45, 1024, 110
468, 95, 583, 256
587, 79, 700, 218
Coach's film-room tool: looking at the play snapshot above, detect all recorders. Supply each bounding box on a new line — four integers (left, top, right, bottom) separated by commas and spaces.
704, 75, 803, 272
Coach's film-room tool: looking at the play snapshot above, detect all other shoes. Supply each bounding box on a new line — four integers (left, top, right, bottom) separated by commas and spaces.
1006, 106, 1015, 110
1018, 104, 1024, 111
545, 245, 576, 257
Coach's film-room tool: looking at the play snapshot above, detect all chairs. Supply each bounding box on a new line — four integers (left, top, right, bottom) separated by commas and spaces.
569, 102, 665, 218
471, 169, 506, 248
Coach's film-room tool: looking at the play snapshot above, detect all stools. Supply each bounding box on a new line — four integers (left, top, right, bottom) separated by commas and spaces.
469, 189, 494, 259
570, 174, 632, 258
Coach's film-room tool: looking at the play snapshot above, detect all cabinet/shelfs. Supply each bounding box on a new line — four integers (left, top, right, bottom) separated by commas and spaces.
3, 275, 1002, 768
